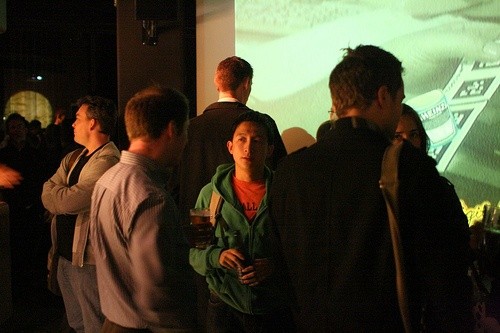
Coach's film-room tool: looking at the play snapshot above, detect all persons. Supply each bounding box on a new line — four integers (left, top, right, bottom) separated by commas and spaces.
91, 88, 214, 333
0, 97, 120, 333
168, 44, 471, 333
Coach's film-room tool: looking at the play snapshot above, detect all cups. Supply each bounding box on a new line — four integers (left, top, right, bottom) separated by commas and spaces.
189, 209, 212, 250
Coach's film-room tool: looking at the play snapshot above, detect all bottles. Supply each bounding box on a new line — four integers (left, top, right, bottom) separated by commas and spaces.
232, 230, 255, 287
